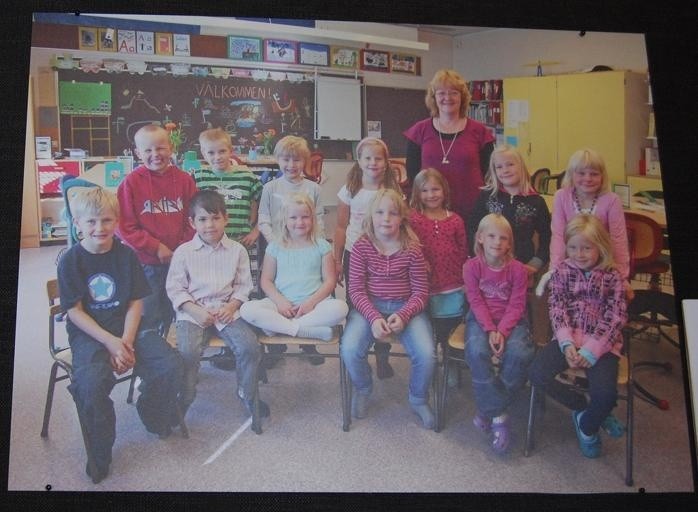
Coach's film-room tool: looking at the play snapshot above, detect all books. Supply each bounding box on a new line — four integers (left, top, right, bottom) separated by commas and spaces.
51, 220, 68, 236
77, 25, 191, 57
64, 148, 85, 157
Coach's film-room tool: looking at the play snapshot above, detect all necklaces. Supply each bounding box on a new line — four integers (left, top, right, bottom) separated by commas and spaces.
436, 117, 464, 163
574, 191, 599, 219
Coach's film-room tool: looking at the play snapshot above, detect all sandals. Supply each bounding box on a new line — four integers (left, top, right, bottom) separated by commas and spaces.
491, 416, 510, 453
603, 413, 626, 437
472, 408, 489, 430
573, 407, 601, 458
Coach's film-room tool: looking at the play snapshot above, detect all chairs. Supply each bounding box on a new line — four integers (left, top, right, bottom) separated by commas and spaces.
545, 170, 568, 196
532, 167, 548, 189
617, 208, 688, 354
38, 275, 640, 491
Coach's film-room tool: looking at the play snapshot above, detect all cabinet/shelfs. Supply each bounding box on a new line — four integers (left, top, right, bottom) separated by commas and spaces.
70, 113, 111, 156
38, 155, 124, 247
464, 80, 506, 150
502, 71, 649, 188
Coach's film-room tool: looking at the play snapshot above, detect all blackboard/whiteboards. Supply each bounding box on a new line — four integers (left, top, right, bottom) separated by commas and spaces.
313, 76, 361, 142
52, 66, 432, 162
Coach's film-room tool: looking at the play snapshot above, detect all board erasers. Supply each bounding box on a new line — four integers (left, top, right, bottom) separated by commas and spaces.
321, 136, 330, 139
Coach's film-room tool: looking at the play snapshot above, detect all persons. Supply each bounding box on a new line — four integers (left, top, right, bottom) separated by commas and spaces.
545, 146, 629, 358
527, 216, 625, 462
117, 124, 197, 327
57, 189, 186, 484
405, 166, 466, 384
343, 190, 440, 431
258, 134, 325, 365
238, 198, 348, 343
467, 143, 574, 329
462, 214, 528, 456
404, 66, 495, 252
163, 191, 269, 420
192, 127, 261, 255
333, 136, 404, 377
